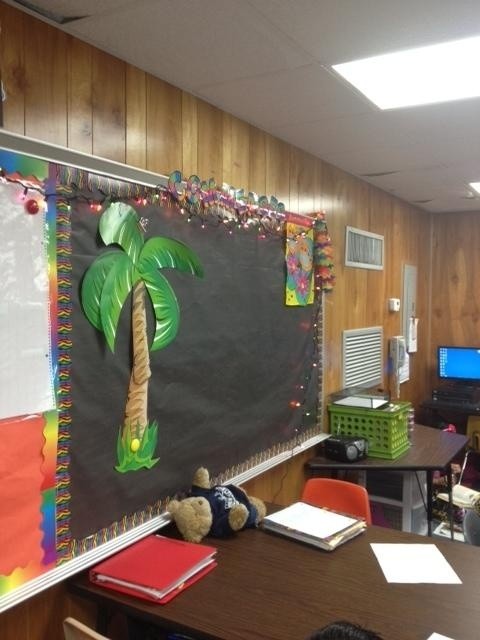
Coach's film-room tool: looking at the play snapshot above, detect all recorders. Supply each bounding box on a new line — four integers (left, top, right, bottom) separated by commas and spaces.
325, 435, 369, 463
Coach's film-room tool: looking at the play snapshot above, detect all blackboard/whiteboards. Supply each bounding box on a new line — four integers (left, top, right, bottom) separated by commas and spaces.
0, 128, 332, 612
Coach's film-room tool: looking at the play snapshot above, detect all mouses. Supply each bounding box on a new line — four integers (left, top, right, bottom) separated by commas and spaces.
476, 408, 480, 411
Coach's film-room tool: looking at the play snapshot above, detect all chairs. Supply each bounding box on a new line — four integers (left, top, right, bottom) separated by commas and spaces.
300, 478, 373, 524
458, 415, 480, 485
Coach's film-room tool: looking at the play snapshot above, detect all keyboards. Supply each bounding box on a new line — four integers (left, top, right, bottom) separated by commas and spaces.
423, 398, 464, 409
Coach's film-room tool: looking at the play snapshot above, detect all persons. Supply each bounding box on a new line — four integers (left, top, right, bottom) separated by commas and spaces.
306, 620, 379, 638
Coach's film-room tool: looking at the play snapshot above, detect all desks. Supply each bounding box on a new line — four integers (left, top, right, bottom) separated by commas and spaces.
63, 501, 479, 640
421, 386, 480, 435
305, 423, 472, 540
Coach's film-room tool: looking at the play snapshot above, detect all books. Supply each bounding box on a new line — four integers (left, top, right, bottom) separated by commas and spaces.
263, 500, 369, 555
432, 519, 466, 542
437, 483, 480, 508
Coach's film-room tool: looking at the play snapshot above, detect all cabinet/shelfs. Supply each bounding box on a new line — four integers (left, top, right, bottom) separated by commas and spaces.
358, 469, 430, 536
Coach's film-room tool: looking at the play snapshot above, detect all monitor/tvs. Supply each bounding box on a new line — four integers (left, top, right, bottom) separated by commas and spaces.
437, 346, 480, 389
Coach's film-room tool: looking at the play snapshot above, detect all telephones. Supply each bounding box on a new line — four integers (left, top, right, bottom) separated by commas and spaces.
389, 335, 406, 371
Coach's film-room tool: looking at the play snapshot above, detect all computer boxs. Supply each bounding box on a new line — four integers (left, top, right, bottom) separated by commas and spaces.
432, 383, 480, 404
